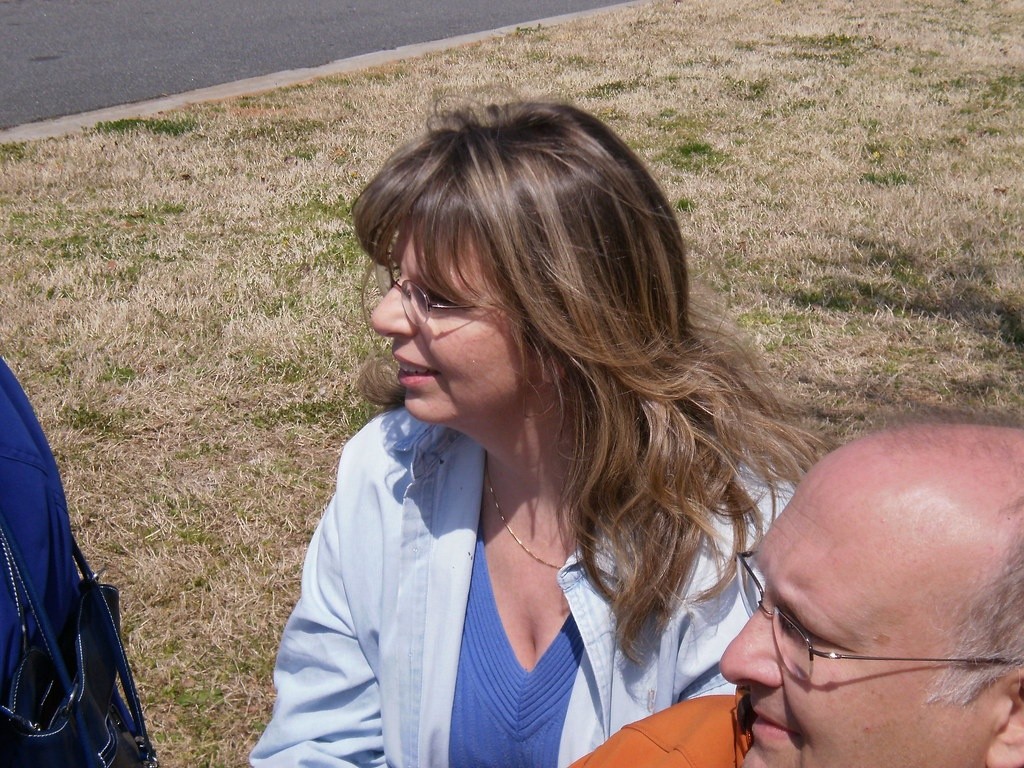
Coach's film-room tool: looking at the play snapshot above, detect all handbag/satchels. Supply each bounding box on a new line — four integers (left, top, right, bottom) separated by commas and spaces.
0, 514, 160, 768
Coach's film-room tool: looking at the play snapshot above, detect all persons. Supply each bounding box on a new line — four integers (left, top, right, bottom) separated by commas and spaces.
247, 102, 801, 767
574, 420, 1024, 768
0, 360, 135, 765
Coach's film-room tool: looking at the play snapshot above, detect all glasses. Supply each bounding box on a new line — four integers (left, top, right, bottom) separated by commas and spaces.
375, 253, 507, 326
734, 550, 1024, 681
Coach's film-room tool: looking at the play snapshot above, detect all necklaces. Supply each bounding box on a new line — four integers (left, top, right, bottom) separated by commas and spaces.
488, 482, 567, 570
